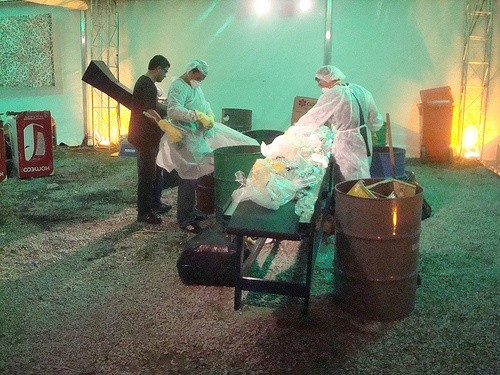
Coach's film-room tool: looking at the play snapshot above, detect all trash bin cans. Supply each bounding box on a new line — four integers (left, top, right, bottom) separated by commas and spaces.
415, 86, 455, 164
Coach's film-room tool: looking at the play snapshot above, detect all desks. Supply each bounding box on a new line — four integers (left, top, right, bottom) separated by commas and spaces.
227, 159, 333, 314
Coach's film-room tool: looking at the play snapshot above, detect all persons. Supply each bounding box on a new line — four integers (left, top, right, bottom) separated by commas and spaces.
166, 59, 216, 235
285, 65, 384, 234
128, 55, 184, 226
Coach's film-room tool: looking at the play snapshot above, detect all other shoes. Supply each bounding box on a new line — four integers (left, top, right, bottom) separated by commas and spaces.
153, 202, 172, 214
181, 222, 202, 233
194, 211, 208, 221
137, 212, 162, 224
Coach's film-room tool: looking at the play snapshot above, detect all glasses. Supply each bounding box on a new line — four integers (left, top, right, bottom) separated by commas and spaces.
161, 66, 168, 73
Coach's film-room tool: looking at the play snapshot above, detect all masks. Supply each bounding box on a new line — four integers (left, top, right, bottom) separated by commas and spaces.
321, 87, 330, 93
190, 80, 200, 89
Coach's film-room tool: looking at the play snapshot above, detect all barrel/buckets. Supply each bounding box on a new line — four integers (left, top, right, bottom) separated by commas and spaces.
371, 123, 386, 146
369, 146, 405, 180
240, 130, 285, 147
213, 145, 265, 223
195, 170, 215, 215
335, 178, 424, 322
221, 108, 252, 132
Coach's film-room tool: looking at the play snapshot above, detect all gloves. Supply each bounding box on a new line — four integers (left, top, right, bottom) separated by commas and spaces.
207, 111, 214, 128
192, 109, 213, 130
158, 119, 183, 143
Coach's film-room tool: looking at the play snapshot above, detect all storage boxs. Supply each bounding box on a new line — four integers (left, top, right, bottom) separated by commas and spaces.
290, 95, 321, 126
7, 109, 58, 179
